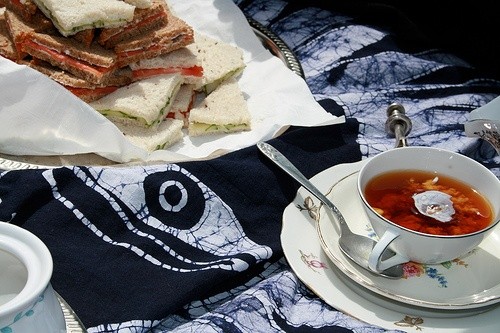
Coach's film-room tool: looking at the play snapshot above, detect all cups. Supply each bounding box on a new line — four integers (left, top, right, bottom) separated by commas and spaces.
357, 146, 500, 272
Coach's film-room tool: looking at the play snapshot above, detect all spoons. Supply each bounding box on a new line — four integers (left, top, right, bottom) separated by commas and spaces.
257, 141, 404, 280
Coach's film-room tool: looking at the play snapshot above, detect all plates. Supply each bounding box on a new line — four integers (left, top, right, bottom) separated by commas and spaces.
0, 16, 300, 171
280, 157, 500, 333
317, 170, 500, 309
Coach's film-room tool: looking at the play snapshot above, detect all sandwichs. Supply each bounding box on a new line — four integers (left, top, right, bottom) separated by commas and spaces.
0, 0, 252, 152
